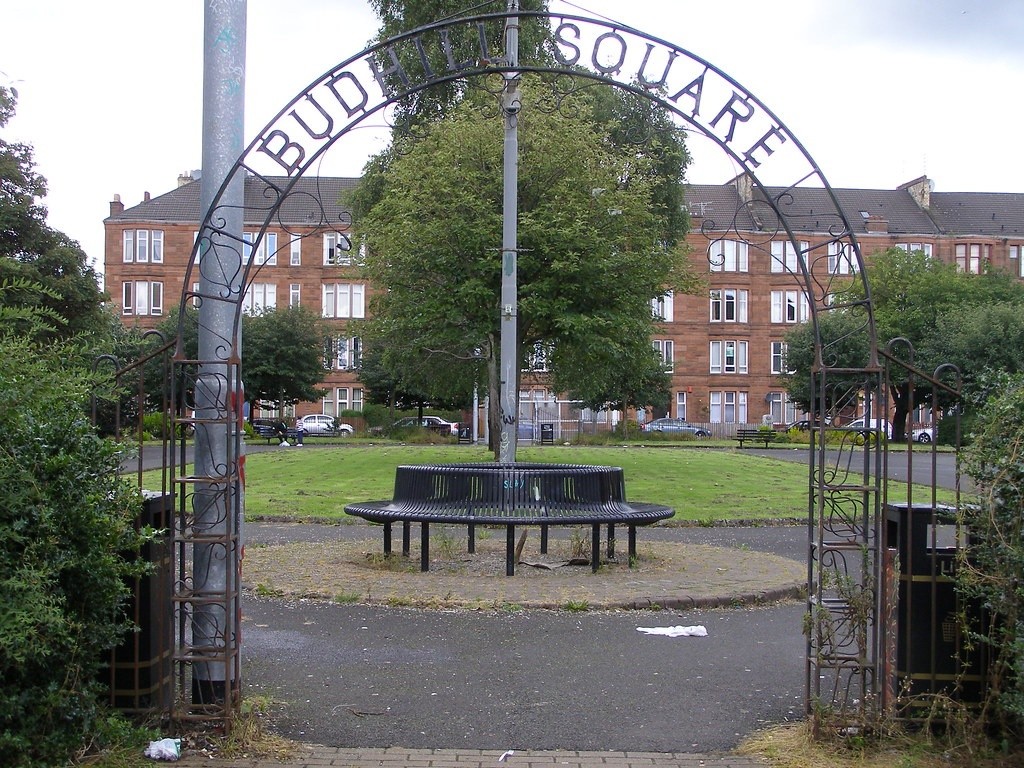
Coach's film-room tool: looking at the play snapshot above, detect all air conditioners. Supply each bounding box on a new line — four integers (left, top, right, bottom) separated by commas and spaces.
762, 414, 773, 429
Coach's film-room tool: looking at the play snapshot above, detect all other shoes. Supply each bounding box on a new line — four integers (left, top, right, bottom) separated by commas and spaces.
296, 443, 303, 446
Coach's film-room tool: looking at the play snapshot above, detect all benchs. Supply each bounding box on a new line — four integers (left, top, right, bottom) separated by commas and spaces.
260, 428, 298, 445
344, 462, 675, 576
732, 429, 777, 449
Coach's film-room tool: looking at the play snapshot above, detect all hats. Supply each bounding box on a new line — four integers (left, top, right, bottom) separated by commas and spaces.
281, 418, 286, 421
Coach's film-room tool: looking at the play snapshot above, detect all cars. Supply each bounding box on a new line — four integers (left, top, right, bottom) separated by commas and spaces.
295, 414, 355, 438
369, 414, 462, 438
252, 419, 276, 437
842, 418, 894, 441
639, 418, 712, 439
904, 425, 937, 443
783, 420, 831, 436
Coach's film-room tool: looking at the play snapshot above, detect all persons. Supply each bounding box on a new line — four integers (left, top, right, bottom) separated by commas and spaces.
296, 415, 304, 446
276, 419, 290, 446
